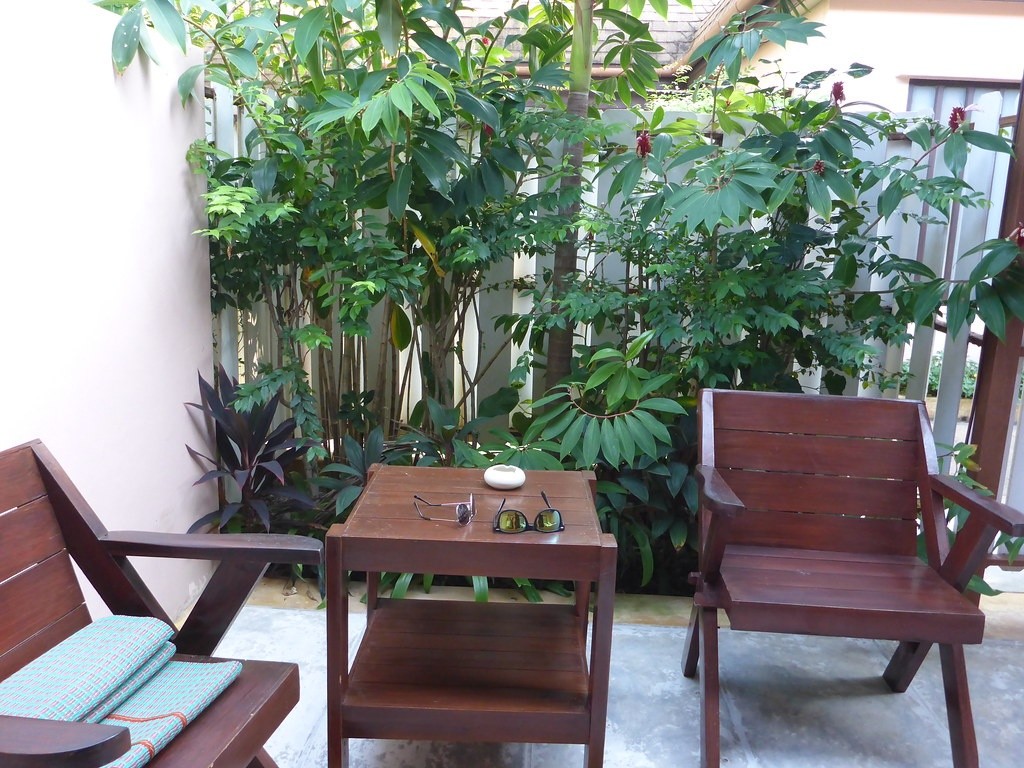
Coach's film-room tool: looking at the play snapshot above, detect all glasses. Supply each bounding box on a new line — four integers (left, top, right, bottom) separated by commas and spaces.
414, 492, 478, 527
493, 491, 565, 534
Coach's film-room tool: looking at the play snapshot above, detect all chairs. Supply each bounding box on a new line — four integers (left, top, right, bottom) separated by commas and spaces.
681, 389, 1024, 768
0, 437, 324, 768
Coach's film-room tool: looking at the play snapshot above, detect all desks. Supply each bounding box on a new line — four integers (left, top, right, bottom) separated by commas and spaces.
324, 464, 618, 768
